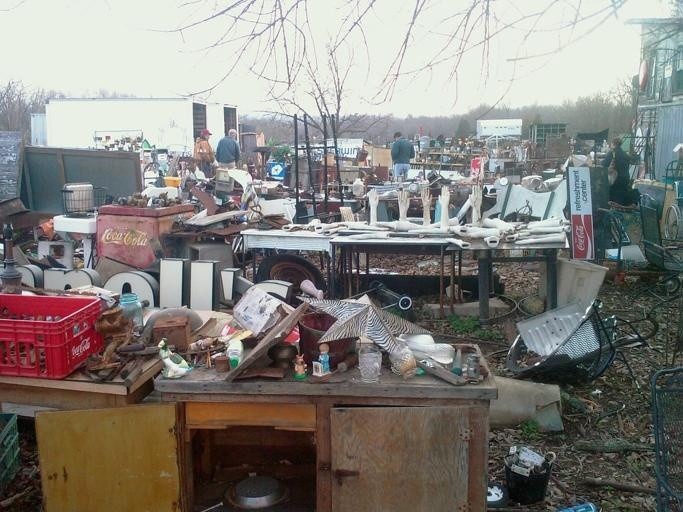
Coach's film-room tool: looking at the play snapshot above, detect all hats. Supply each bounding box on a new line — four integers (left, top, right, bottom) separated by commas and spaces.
607, 158, 619, 185
200, 129, 212, 136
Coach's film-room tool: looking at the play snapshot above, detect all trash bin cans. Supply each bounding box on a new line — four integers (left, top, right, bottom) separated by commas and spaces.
505, 452, 553, 504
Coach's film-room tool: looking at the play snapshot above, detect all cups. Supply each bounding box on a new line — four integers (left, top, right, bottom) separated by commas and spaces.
388, 342, 418, 381
357, 345, 382, 383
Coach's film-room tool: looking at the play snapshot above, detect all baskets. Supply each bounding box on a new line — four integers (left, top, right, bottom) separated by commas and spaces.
0, 294, 104, 379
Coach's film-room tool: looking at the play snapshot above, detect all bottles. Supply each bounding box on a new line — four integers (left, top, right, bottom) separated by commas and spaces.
118, 293, 144, 334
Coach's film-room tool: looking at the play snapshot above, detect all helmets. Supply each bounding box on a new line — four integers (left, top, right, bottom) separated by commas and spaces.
164, 176, 182, 187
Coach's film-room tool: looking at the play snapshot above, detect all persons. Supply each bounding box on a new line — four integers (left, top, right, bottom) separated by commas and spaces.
599, 137, 631, 205
195, 129, 216, 189
216, 129, 240, 192
391, 131, 415, 180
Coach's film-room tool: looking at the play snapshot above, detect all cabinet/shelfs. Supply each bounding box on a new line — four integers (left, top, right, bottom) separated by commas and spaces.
35, 343, 498, 512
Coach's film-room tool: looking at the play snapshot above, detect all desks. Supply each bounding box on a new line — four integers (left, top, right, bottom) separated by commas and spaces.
0, 354, 164, 410
241, 190, 569, 327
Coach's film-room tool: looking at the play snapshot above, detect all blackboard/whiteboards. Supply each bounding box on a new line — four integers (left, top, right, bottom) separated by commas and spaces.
25, 146, 145, 213
1, 131, 23, 196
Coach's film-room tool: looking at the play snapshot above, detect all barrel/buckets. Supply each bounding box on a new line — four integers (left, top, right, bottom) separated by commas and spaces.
297, 310, 357, 370
